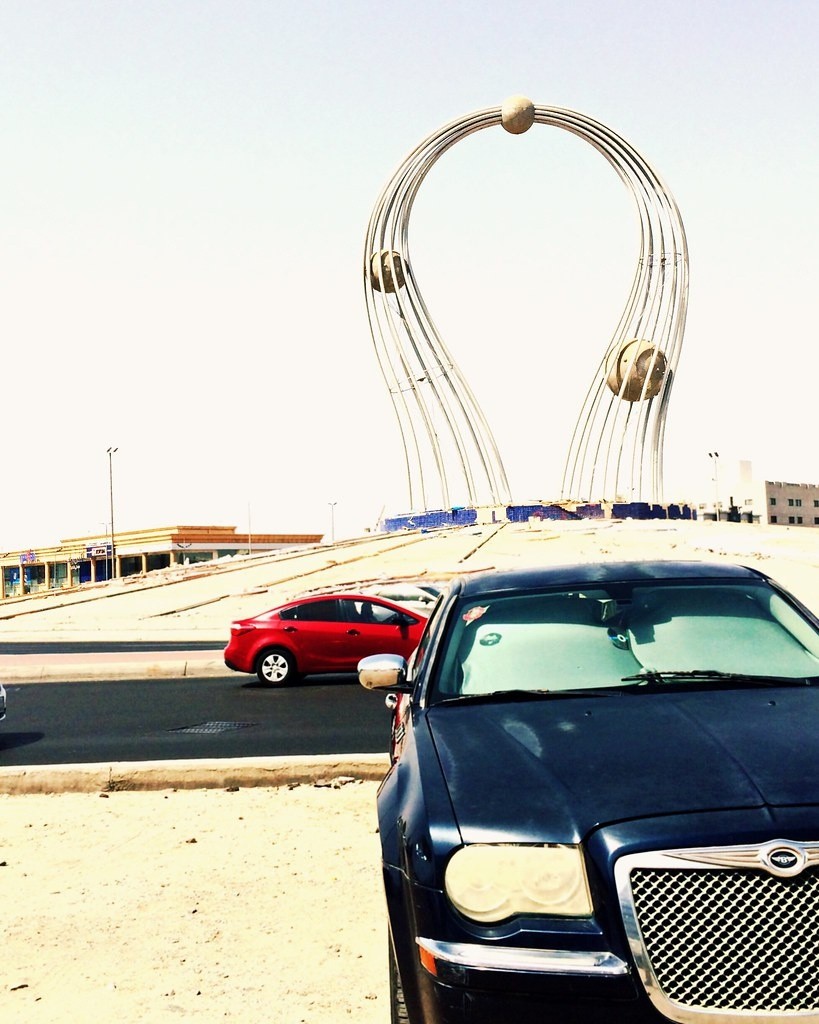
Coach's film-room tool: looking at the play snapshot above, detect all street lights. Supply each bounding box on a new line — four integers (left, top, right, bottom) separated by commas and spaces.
328, 502, 337, 541
107, 447, 119, 578
709, 452, 719, 521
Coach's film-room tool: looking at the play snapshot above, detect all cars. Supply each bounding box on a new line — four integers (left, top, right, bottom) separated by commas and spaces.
358, 560, 819, 1023
225, 594, 429, 688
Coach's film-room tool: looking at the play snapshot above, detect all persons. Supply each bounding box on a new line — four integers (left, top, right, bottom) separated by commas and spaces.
359, 602, 396, 624
